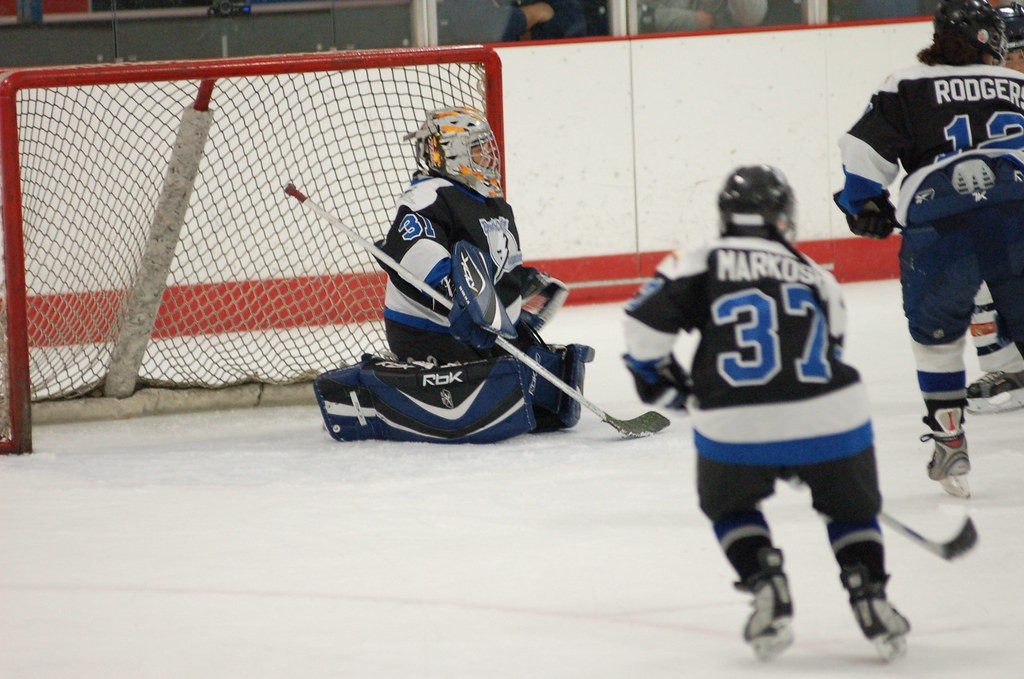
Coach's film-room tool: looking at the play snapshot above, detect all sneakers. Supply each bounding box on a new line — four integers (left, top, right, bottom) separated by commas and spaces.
922, 408, 974, 499
963, 366, 1024, 414
840, 565, 909, 661
735, 546, 793, 660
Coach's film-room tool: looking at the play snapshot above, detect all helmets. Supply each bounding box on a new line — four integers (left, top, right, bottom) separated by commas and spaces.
717, 164, 796, 244
413, 105, 503, 199
994, 2, 1024, 51
933, 0, 1008, 69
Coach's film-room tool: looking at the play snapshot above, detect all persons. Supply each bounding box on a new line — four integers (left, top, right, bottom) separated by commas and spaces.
833, 0, 1024, 500
375, 105, 569, 367
436, 0, 768, 46
621, 166, 914, 662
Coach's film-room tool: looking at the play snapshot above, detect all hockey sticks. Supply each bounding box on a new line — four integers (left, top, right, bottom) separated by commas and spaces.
283, 183, 672, 440
878, 514, 979, 562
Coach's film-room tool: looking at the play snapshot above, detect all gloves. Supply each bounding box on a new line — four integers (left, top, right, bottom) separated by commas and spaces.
834, 187, 897, 241
633, 353, 691, 410
